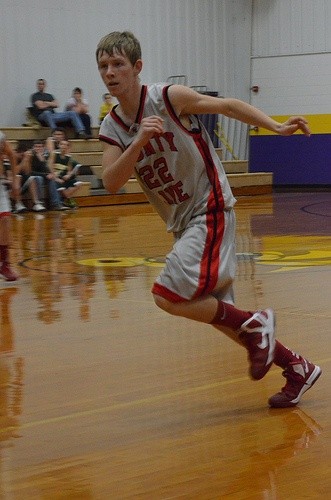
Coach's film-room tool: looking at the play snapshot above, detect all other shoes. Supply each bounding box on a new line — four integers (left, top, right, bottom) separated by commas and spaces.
55, 204, 68, 211
79, 133, 91, 140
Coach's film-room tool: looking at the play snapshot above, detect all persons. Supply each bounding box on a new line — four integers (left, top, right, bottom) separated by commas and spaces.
64, 87, 92, 139
0, 127, 83, 281
96, 32, 322, 407
31, 79, 92, 139
98, 93, 116, 125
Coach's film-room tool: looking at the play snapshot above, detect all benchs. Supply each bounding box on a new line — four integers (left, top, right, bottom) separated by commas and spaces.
0, 127, 272, 206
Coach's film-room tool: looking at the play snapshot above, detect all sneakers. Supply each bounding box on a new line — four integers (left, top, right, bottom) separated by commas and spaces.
0, 263, 18, 281
238, 309, 277, 379
267, 357, 322, 408
33, 202, 46, 212
15, 202, 25, 212
